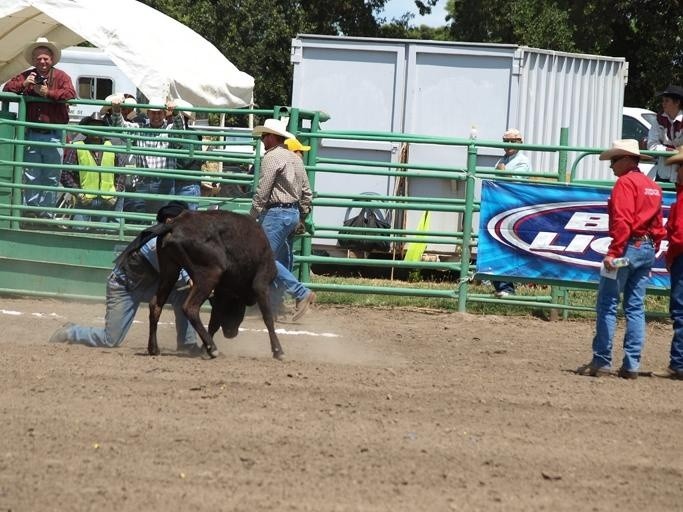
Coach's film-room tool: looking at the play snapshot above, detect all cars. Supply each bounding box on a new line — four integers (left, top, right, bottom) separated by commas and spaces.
620, 103, 658, 175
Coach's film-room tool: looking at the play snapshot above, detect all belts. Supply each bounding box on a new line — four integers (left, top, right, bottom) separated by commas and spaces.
632, 234, 653, 241
268, 202, 299, 209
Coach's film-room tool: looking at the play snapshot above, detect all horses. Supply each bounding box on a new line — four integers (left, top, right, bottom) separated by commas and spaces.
111, 208, 288, 364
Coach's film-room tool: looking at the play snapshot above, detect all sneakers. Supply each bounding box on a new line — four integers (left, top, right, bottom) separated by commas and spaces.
177, 342, 204, 357
48, 321, 75, 343
291, 290, 317, 322
576, 362, 610, 378
650, 365, 683, 380
617, 367, 638, 380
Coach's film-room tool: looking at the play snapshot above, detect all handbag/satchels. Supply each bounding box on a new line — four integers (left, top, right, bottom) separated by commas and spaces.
338, 199, 392, 252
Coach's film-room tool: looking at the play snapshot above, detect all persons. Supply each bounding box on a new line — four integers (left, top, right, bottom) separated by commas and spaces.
574, 139, 667, 379
249, 119, 317, 321
652, 146, 682, 378
275, 139, 310, 312
647, 85, 682, 182
0, 37, 78, 228
60, 88, 201, 234
489, 128, 532, 298
47, 199, 202, 357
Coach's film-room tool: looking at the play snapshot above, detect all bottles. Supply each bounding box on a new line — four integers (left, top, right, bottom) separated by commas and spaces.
612, 257, 629, 268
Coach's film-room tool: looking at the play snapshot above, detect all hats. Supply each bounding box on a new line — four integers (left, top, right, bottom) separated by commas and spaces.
24, 37, 62, 67
663, 145, 683, 166
99, 90, 138, 114
253, 118, 297, 141
173, 98, 196, 121
503, 128, 524, 144
138, 96, 168, 115
284, 138, 311, 153
654, 85, 683, 99
156, 199, 190, 223
79, 112, 110, 136
599, 138, 654, 161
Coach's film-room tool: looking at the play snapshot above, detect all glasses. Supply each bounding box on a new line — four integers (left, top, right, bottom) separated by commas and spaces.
503, 139, 520, 143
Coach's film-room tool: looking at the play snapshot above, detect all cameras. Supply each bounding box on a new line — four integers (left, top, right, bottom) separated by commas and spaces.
35, 75, 47, 85
